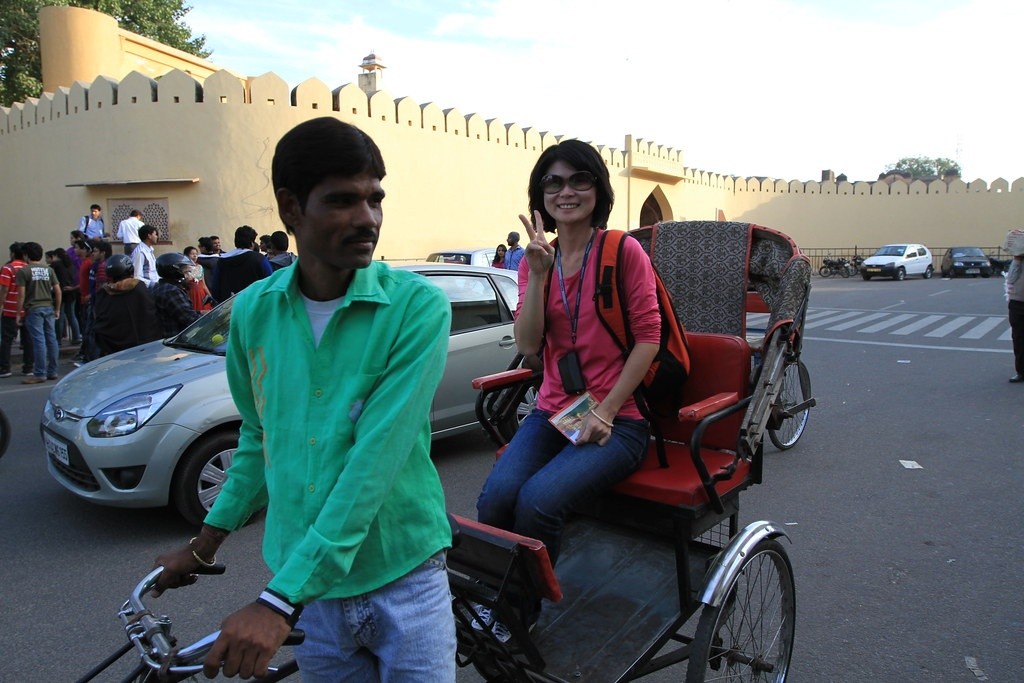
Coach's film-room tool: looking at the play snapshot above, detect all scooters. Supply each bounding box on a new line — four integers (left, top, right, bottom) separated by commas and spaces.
819, 253, 864, 279
986, 251, 1012, 275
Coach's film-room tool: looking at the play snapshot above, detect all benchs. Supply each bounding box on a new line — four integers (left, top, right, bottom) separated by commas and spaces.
473, 337, 754, 507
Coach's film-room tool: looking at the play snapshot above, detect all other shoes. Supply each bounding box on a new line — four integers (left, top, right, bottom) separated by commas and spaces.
20, 368, 33, 375
73, 362, 85, 367
71, 334, 83, 345
21, 377, 46, 383
47, 372, 58, 380
57, 336, 64, 345
1009, 374, 1024, 382
0, 367, 12, 378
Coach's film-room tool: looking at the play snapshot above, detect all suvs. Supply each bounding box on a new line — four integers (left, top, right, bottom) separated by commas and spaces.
862, 244, 934, 281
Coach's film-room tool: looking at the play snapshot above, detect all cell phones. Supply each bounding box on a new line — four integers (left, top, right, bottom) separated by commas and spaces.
556, 350, 586, 395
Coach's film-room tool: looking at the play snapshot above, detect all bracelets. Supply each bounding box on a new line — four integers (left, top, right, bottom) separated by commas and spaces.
190, 535, 216, 566
591, 409, 613, 427
256, 588, 304, 629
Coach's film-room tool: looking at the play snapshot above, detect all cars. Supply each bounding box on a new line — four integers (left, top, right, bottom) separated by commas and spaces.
941, 246, 992, 278
39, 264, 544, 530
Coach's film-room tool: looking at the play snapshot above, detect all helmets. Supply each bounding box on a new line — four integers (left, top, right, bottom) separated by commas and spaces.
104, 255, 135, 284
157, 253, 196, 291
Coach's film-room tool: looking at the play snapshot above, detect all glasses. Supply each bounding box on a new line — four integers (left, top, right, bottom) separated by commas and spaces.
540, 169, 595, 195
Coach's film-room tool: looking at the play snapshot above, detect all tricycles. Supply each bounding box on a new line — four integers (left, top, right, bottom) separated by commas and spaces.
75, 219, 815, 683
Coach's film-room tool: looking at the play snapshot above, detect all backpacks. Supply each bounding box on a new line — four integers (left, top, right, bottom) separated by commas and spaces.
537, 229, 692, 425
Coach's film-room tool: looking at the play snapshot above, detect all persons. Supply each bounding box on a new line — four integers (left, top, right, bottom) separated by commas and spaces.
463, 139, 667, 647
1006, 254, 1024, 382
0, 203, 525, 385
148, 118, 459, 683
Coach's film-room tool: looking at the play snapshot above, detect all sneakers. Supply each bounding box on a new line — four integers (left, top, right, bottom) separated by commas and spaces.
471, 605, 495, 630
491, 620, 536, 644
